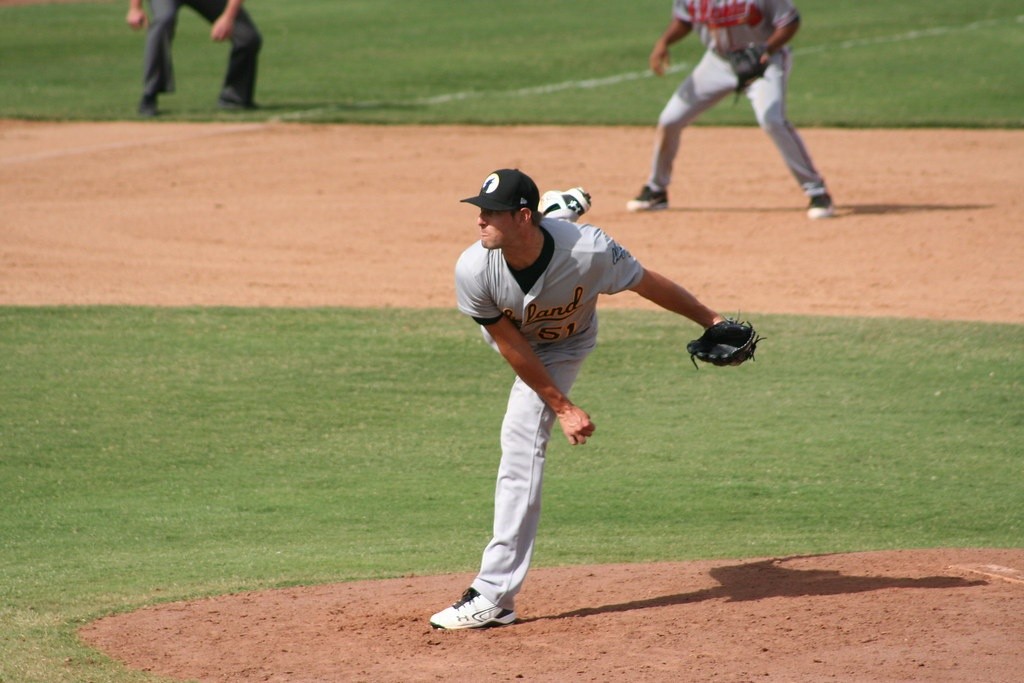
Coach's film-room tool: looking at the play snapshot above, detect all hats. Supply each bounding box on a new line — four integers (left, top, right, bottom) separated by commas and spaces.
460, 168, 540, 211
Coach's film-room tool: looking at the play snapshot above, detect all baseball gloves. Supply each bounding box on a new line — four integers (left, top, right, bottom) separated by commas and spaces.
686, 307, 769, 373
726, 41, 773, 106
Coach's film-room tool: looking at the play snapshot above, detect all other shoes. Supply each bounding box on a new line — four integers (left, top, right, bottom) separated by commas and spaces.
138, 94, 158, 115
217, 97, 256, 110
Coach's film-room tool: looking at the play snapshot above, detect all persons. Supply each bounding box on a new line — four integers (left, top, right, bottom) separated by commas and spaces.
127, 0, 263, 116
627, 0, 834, 219
430, 168, 758, 630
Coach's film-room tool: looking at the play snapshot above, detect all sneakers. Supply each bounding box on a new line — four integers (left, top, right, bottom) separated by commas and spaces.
806, 193, 833, 219
541, 186, 592, 215
430, 587, 516, 629
627, 185, 668, 210
